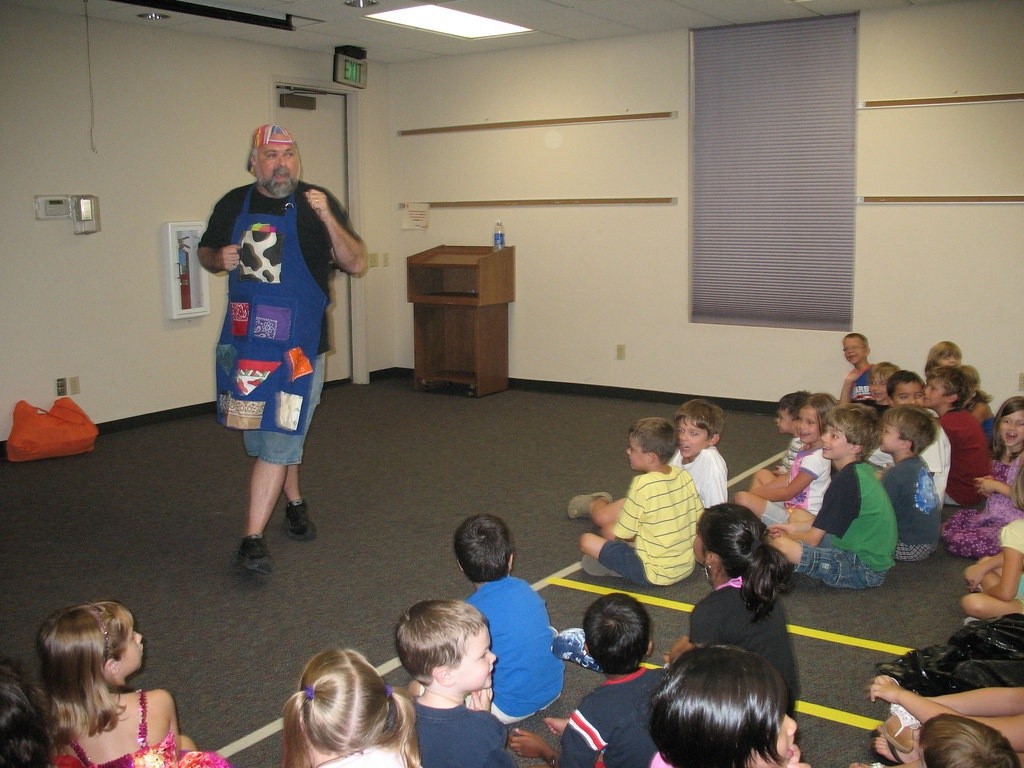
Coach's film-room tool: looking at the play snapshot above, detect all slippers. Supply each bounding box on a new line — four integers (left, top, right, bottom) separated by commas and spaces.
868, 726, 906, 766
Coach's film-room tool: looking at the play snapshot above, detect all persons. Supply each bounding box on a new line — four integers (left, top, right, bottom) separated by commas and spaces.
197, 125, 364, 575
281, 502, 814, 768
850, 395, 1024, 768
0, 600, 235, 768
567, 333, 1004, 589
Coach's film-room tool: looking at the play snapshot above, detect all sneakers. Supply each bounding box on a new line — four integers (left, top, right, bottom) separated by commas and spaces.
285, 498, 318, 540
238, 533, 273, 578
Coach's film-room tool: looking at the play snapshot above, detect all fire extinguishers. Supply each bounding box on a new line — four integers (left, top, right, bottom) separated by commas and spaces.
179, 236, 191, 309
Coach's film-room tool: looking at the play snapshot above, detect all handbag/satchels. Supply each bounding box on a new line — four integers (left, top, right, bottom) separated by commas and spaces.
5, 397, 99, 463
873, 613, 1024, 698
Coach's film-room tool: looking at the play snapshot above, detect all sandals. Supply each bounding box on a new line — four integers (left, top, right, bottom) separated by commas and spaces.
582, 552, 623, 577
567, 491, 613, 519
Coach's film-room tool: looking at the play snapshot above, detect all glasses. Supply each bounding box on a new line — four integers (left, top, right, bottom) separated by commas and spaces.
843, 345, 865, 351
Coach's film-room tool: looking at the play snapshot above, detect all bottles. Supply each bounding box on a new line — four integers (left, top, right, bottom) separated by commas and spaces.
493, 219, 505, 253
